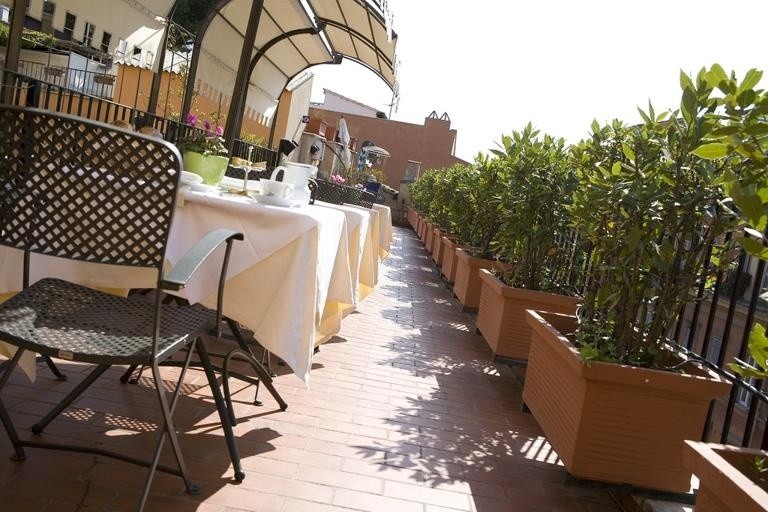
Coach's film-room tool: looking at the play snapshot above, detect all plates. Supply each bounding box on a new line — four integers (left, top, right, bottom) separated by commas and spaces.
252, 194, 301, 208
179, 171, 222, 192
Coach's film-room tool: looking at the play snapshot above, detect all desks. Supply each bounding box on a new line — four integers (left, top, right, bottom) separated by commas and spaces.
3, 157, 393, 483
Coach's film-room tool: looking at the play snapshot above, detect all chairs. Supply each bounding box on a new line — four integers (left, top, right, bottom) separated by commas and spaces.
0, 101, 244, 511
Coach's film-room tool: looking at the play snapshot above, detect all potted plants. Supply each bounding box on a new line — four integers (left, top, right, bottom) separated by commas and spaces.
402, 61, 768, 511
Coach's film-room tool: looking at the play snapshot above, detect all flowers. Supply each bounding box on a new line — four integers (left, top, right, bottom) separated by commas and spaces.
185, 109, 227, 155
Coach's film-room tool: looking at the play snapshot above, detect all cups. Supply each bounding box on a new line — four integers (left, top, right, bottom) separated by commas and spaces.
260, 162, 317, 207
233, 157, 266, 168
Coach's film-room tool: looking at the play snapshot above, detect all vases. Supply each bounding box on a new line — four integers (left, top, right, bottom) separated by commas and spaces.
182, 149, 231, 186
